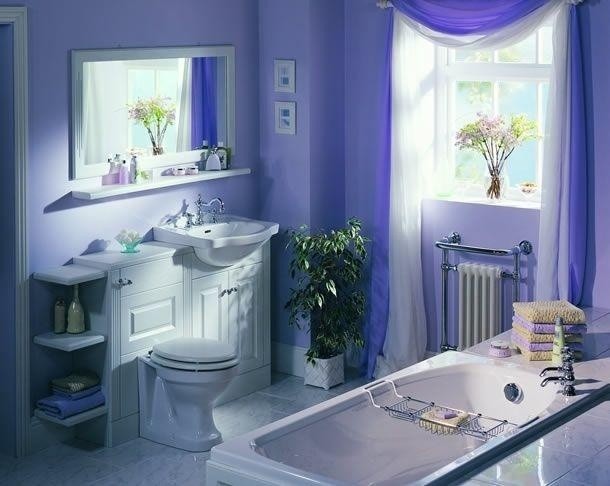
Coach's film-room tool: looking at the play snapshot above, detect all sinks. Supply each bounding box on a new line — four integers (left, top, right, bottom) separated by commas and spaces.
152, 214, 280, 267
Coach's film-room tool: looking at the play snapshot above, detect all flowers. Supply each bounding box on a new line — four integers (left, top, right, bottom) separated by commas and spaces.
453, 113, 541, 198
126, 96, 177, 155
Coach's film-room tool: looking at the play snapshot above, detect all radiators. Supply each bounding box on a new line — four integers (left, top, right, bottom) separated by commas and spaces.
456, 263, 501, 350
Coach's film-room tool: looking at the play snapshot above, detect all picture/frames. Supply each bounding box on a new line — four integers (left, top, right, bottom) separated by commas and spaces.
274, 60, 296, 93
274, 102, 296, 135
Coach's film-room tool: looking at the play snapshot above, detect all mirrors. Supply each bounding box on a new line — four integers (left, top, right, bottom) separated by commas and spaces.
71, 45, 236, 179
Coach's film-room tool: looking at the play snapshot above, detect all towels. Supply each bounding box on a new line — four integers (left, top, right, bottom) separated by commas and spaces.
512, 316, 588, 334
511, 335, 582, 352
50, 371, 99, 393
512, 324, 584, 343
52, 386, 101, 400
512, 343, 582, 362
513, 300, 585, 324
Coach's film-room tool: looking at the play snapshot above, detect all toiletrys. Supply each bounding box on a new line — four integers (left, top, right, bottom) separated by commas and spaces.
120, 160, 129, 185
174, 167, 185, 176
129, 155, 137, 182
201, 140, 210, 149
189, 165, 199, 175
216, 142, 230, 171
206, 146, 221, 171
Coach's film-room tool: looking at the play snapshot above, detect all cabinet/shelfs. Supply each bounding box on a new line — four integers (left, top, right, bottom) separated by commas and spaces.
31, 236, 272, 451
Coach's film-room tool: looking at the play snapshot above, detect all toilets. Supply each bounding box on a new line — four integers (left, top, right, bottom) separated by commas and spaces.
137, 336, 241, 451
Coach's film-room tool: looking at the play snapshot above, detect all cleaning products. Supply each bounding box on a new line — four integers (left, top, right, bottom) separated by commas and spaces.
205, 148, 222, 171
213, 141, 230, 170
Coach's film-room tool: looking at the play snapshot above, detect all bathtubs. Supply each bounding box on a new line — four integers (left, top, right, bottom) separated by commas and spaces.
205, 349, 610, 485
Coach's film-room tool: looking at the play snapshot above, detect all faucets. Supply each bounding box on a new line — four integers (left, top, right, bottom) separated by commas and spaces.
539, 347, 578, 397
182, 193, 224, 228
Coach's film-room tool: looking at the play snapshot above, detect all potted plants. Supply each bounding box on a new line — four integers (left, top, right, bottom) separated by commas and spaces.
280, 216, 373, 391
